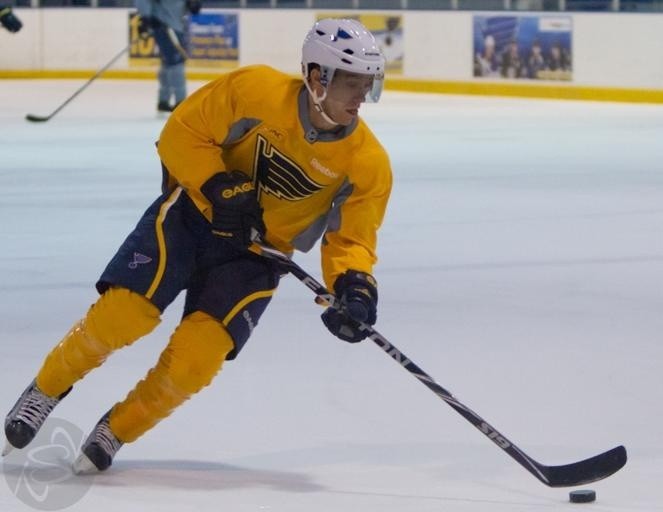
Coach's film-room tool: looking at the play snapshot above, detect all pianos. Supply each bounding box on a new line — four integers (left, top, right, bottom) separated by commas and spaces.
250, 228, 627, 485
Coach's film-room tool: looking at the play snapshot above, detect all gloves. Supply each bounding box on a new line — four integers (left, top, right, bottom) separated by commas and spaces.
187, 0, 201, 12
137, 15, 153, 32
0, 7, 21, 32
201, 170, 266, 249
321, 270, 378, 343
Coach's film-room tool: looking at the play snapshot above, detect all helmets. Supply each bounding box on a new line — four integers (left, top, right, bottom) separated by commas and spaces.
301, 19, 384, 103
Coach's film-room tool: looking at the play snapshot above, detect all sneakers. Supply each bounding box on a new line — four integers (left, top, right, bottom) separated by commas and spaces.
82, 408, 124, 471
4, 378, 73, 449
157, 102, 176, 111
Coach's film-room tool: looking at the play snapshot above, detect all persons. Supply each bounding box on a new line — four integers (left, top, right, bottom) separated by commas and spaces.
136, 0, 202, 112
473, 34, 571, 79
382, 36, 395, 63
0, 0, 22, 32
5, 18, 393, 474
